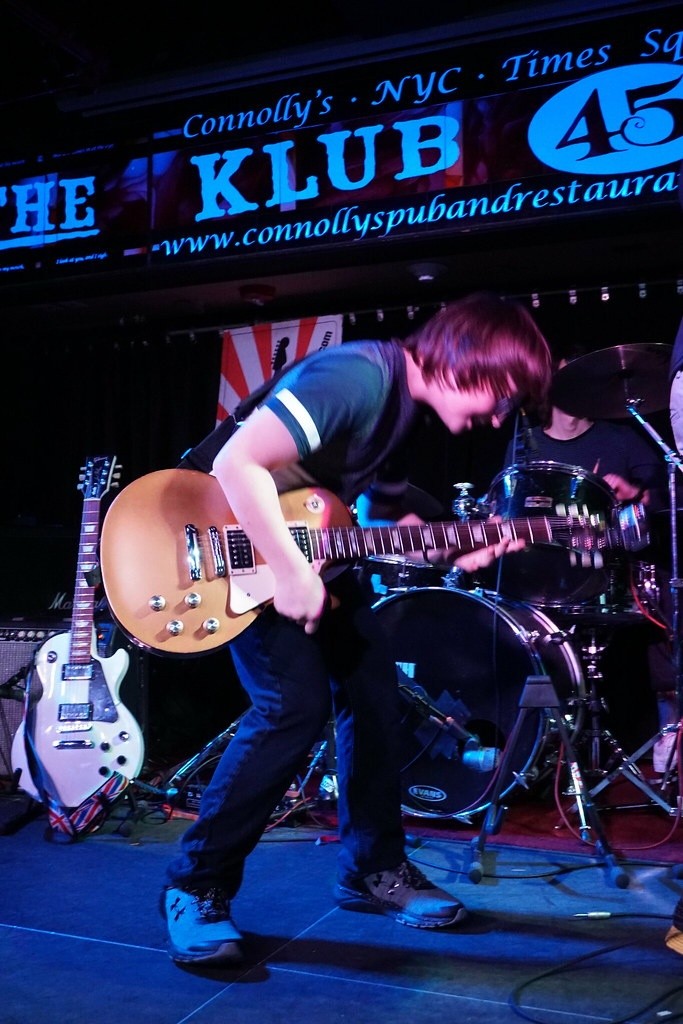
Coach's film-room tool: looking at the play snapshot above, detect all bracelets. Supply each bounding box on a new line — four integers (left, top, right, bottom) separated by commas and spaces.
629, 487, 648, 505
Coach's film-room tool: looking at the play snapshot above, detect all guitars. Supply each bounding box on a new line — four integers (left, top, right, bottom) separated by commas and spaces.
101, 466, 609, 656
10, 456, 147, 809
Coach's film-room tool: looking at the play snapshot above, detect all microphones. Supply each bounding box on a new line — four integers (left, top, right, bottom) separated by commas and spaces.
462, 738, 502, 772
0, 685, 27, 703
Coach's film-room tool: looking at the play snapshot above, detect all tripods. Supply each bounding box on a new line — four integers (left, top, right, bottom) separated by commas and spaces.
518, 396, 683, 830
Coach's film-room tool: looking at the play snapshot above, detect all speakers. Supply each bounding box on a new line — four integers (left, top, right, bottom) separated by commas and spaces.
0, 618, 149, 781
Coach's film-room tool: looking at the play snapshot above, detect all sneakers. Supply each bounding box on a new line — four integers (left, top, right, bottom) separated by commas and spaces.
160, 883, 243, 962
335, 866, 466, 928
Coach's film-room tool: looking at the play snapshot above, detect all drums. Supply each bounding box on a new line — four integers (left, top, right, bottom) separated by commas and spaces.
341, 582, 584, 819
468, 459, 623, 608
359, 552, 437, 592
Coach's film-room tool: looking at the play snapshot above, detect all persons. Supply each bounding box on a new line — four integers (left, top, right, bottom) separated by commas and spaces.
503, 319, 683, 955
157, 295, 554, 967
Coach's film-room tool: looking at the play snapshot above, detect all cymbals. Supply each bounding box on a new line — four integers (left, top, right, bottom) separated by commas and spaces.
548, 341, 675, 420
